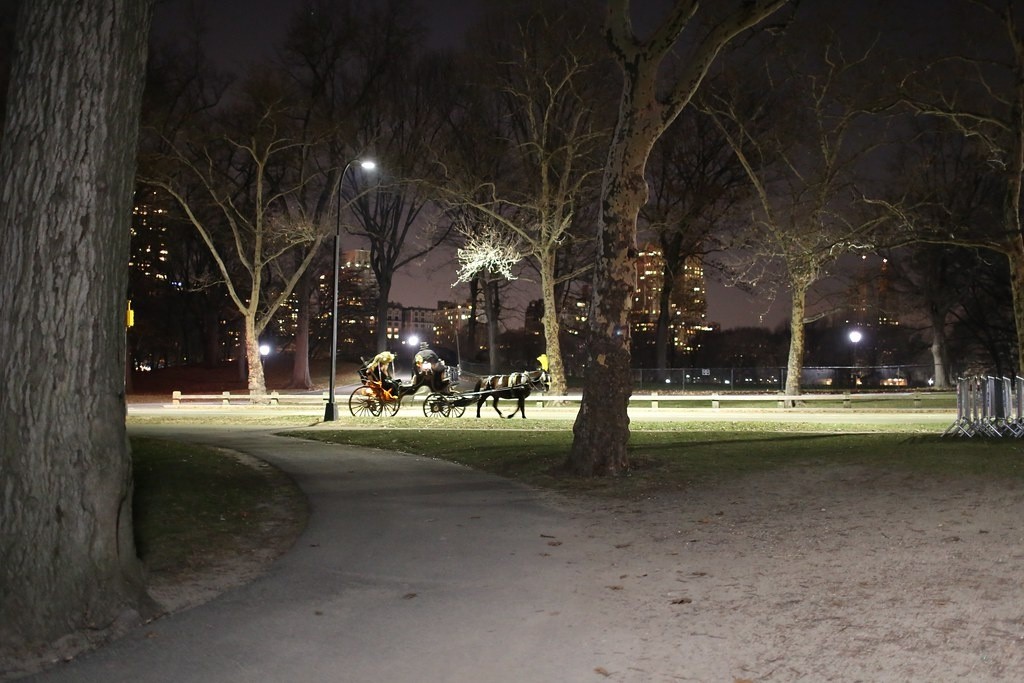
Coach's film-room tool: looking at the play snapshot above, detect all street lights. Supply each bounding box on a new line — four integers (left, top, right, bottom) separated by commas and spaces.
259, 344, 270, 371
406, 334, 420, 363
848, 330, 862, 389
322, 157, 377, 421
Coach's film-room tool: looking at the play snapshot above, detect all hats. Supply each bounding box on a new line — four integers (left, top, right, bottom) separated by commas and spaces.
419, 342, 429, 347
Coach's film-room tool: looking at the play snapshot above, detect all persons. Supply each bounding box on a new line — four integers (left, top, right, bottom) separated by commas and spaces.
368, 351, 398, 398
537, 354, 549, 393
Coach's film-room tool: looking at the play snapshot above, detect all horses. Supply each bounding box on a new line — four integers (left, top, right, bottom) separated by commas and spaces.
472, 368, 553, 419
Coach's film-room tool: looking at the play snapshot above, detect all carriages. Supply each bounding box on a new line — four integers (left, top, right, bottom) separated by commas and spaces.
348, 351, 552, 419
